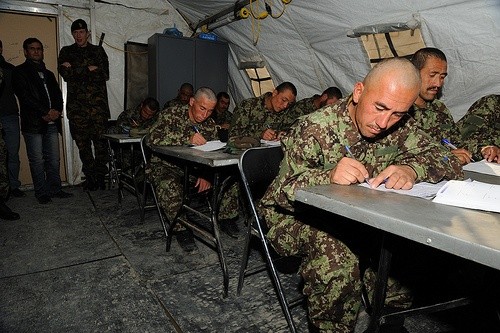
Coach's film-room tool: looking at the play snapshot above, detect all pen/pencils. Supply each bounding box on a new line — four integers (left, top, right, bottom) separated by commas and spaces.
442, 138, 474, 162
345, 146, 371, 185
130, 117, 138, 125
267, 125, 276, 136
194, 126, 200, 134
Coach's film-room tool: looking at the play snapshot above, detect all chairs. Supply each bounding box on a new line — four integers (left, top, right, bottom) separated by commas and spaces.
237, 144, 307, 333
105, 123, 126, 201
139, 132, 171, 238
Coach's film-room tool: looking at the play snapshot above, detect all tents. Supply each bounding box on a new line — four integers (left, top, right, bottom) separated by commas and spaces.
0, 0, 500, 191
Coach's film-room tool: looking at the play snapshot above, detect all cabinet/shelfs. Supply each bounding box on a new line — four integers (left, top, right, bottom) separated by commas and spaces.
148, 34, 229, 119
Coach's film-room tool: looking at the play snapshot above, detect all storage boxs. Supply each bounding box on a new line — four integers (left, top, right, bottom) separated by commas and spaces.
199, 32, 217, 41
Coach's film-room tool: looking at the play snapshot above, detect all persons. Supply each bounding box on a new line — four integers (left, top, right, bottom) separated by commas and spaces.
10, 38, 73, 204
228, 80, 297, 140
257, 56, 464, 333
162, 82, 194, 110
144, 88, 240, 253
210, 92, 233, 143
0, 40, 26, 220
287, 87, 342, 121
456, 94, 500, 163
407, 47, 473, 167
58, 18, 109, 190
116, 97, 160, 181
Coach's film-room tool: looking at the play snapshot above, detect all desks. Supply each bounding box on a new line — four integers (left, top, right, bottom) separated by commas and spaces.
102, 131, 157, 224
150, 139, 281, 298
293, 159, 500, 333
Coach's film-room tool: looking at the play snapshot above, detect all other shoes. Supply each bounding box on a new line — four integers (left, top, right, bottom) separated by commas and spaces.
35, 190, 53, 205
176, 229, 199, 255
53, 190, 73, 198
88, 174, 106, 190
11, 190, 26, 198
0, 206, 20, 220
220, 221, 245, 240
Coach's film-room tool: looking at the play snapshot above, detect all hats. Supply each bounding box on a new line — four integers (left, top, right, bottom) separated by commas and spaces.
71, 19, 87, 31
